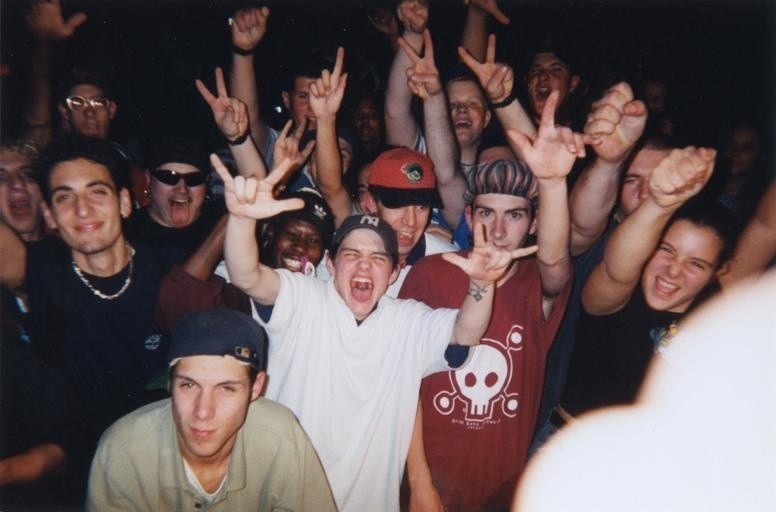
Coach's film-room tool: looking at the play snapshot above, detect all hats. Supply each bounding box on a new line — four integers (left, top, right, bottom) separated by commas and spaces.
368, 147, 445, 208
275, 192, 335, 243
145, 141, 207, 172
329, 214, 398, 262
149, 309, 268, 371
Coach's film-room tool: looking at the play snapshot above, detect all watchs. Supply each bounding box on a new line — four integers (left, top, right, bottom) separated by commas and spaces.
224, 130, 250, 146
230, 45, 261, 55
490, 94, 517, 109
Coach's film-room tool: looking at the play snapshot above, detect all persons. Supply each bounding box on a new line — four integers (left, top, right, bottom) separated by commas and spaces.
210, 154, 539, 512
308, 48, 461, 248
396, 28, 520, 252
0, 140, 49, 241
84, 307, 338, 512
0, 134, 166, 429
463, 1, 583, 132
394, 88, 602, 512
385, 0, 491, 193
529, 145, 774, 472
460, 35, 776, 258
348, 91, 381, 153
0, 286, 96, 512
155, 175, 333, 336
227, 6, 326, 180
362, 145, 463, 263
195, 66, 360, 199
123, 140, 225, 271
20, 0, 117, 139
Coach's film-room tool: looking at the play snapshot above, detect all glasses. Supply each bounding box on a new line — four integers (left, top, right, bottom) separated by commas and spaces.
154, 171, 206, 187
65, 92, 110, 112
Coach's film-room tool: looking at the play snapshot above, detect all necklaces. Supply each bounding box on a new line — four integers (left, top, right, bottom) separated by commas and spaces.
70, 241, 134, 300
